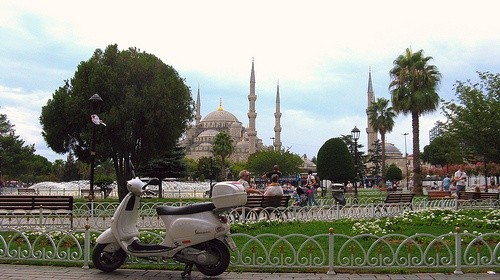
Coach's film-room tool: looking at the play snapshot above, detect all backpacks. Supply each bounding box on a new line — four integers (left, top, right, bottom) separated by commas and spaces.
311, 175, 320, 188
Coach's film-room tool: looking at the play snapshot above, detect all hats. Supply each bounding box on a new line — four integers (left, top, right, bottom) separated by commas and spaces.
239, 170, 249, 175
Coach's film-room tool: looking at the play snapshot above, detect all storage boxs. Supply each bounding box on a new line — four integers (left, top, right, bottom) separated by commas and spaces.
211, 182, 247, 208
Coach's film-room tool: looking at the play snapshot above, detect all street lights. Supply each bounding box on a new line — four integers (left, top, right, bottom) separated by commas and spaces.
401, 132, 410, 189
351, 126, 360, 204
87, 93, 105, 217
208, 148, 213, 199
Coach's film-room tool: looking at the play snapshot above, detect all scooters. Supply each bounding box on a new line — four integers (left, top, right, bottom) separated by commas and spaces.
92, 161, 247, 278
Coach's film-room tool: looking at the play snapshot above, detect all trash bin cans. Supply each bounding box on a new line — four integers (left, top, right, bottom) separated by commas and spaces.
331, 184, 346, 205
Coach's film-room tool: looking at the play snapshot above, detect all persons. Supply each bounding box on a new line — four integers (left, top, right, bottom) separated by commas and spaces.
262, 165, 282, 182
284, 182, 307, 207
472, 187, 481, 200
304, 170, 318, 206
431, 182, 437, 190
263, 174, 283, 196
490, 178, 495, 191
237, 170, 261, 195
391, 180, 400, 189
454, 165, 467, 195
443, 174, 450, 191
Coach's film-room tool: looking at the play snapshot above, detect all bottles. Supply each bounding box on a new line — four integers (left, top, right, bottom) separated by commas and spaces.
316, 187, 322, 197
392, 181, 397, 191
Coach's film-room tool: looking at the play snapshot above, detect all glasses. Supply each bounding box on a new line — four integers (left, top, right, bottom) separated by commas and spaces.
245, 175, 249, 176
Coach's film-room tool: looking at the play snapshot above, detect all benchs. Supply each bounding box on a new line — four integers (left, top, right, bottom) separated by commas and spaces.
428, 191, 454, 200
0, 196, 73, 230
374, 194, 415, 213
248, 196, 291, 219
457, 192, 499, 209
18, 189, 39, 194
